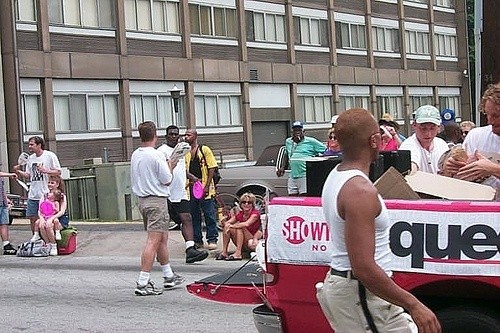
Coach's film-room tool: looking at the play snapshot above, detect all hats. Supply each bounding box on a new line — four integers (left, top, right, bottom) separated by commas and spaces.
292, 120, 303, 129
441, 108, 455, 126
331, 115, 340, 124
415, 105, 440, 127
378, 113, 400, 130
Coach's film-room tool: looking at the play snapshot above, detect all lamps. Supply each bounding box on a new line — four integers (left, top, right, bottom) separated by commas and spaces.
167, 84, 183, 113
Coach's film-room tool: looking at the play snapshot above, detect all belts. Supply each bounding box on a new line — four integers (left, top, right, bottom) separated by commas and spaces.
331, 268, 357, 280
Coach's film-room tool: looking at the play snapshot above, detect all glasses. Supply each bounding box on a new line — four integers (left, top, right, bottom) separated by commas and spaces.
241, 201, 252, 204
461, 131, 469, 135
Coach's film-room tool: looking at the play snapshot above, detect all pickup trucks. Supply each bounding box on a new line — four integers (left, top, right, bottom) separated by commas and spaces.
186, 196, 500, 333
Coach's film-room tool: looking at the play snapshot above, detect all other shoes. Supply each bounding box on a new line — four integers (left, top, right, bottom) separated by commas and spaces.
162, 269, 186, 288
208, 243, 219, 250
185, 244, 210, 263
3, 243, 17, 255
135, 279, 163, 296
46, 243, 58, 256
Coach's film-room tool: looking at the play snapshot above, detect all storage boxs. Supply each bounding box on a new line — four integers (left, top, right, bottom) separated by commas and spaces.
54, 226, 77, 254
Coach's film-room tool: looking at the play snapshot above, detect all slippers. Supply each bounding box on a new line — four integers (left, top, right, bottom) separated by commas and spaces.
215, 253, 243, 261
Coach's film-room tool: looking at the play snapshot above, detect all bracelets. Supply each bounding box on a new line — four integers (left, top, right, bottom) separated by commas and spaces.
392, 133, 396, 136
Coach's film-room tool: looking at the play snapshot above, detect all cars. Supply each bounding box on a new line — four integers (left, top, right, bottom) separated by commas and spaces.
215, 144, 292, 214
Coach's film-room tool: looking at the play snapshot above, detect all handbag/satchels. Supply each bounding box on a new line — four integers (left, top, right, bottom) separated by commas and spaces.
16, 241, 43, 257
200, 144, 221, 183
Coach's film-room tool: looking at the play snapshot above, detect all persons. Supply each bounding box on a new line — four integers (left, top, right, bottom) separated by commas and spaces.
0, 136, 69, 257
247, 192, 278, 251
380, 84, 500, 201
216, 193, 263, 261
278, 120, 326, 198
315, 107, 441, 333
130, 121, 219, 296
323, 115, 343, 157
